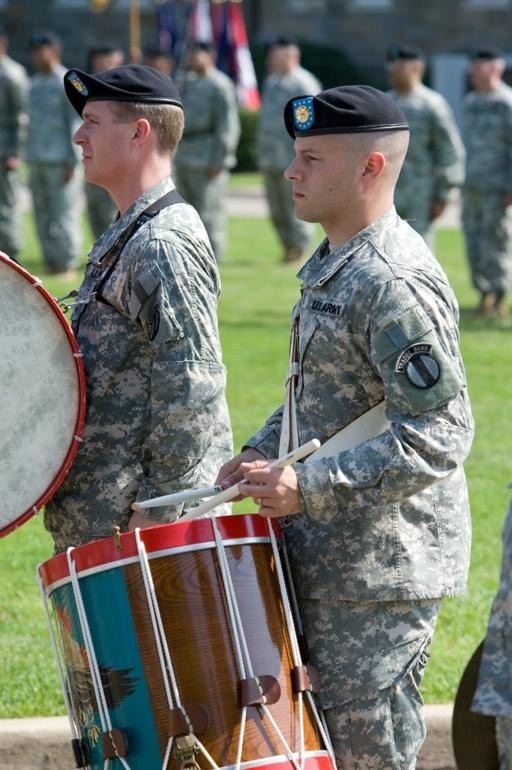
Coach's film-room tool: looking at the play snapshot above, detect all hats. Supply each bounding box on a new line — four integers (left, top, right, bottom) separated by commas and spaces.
388, 43, 424, 62
63, 65, 184, 117
283, 85, 411, 139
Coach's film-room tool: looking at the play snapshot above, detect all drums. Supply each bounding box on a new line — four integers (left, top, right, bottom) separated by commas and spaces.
39, 514, 337, 770
1, 250, 90, 536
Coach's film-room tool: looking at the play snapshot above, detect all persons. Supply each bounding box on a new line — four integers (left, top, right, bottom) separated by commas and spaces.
216, 78, 475, 769
39, 63, 241, 553
257, 37, 320, 263
0, 32, 31, 255
459, 48, 512, 314
87, 45, 125, 238
382, 46, 466, 245
467, 477, 512, 768
154, 42, 238, 258
30, 32, 85, 278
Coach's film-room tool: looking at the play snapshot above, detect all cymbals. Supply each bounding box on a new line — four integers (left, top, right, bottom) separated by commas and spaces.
452, 639, 501, 770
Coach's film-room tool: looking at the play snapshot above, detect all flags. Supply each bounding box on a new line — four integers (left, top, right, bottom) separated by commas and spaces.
194, 1, 264, 115
